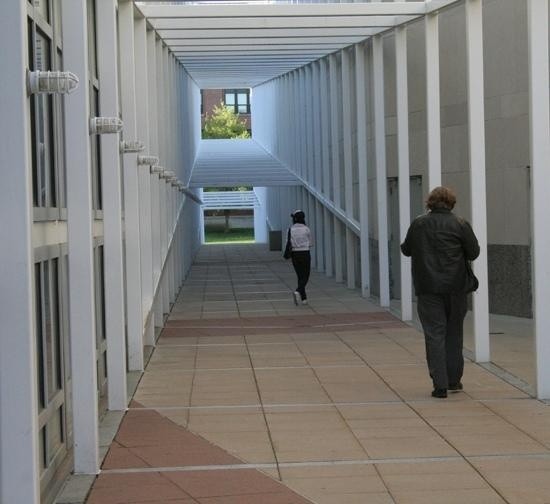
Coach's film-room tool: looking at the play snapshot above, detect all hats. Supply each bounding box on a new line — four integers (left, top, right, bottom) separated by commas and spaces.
290, 210, 305, 218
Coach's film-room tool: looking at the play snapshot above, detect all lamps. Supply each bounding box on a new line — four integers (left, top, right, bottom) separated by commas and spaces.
23, 68, 82, 101
120, 139, 175, 186
88, 114, 124, 140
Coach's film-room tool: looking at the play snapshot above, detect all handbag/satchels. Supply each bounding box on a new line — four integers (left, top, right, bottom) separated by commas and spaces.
284, 240, 292, 260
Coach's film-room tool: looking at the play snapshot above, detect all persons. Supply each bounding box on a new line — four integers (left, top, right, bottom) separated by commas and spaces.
399, 185, 482, 399
283, 208, 314, 306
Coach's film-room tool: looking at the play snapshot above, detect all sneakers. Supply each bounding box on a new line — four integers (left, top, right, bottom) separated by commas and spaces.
432, 388, 447, 398
447, 382, 463, 390
293, 291, 301, 306
